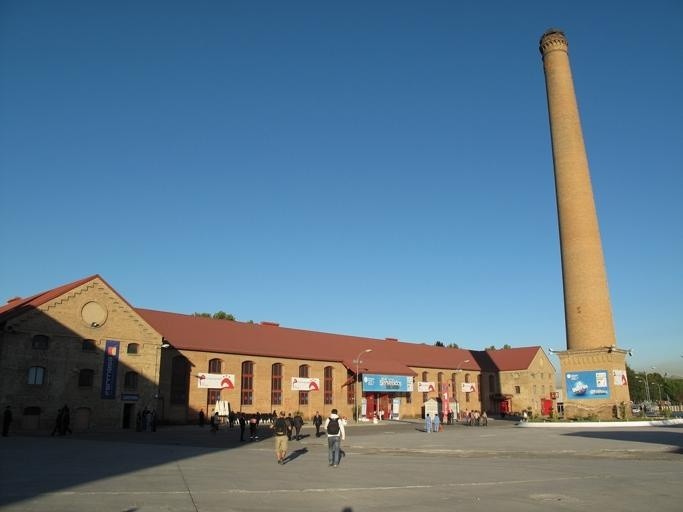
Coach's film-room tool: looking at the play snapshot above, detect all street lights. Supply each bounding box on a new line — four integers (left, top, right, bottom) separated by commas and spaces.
355, 348, 373, 423
633, 366, 664, 402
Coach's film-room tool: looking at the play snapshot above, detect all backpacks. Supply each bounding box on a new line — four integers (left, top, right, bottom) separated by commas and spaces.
327, 416, 341, 435
274, 418, 286, 435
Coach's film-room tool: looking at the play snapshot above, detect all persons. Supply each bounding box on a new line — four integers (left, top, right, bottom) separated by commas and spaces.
136, 405, 157, 432
523, 408, 528, 422
50, 403, 72, 437
425, 407, 487, 434
2, 405, 13, 438
199, 408, 345, 468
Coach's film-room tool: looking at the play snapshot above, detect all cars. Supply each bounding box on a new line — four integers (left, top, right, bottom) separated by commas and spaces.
631, 406, 640, 413
